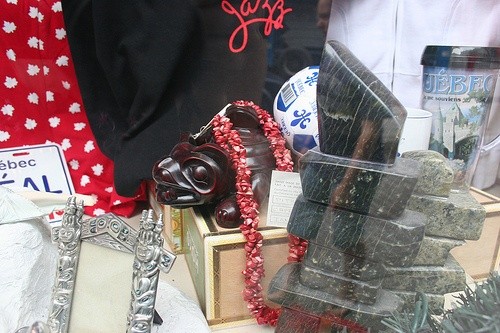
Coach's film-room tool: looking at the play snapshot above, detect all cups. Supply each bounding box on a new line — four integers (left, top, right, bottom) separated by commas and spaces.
394, 106, 433, 162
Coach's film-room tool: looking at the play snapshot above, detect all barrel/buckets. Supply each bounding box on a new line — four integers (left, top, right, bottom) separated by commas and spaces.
419, 44, 500, 192
